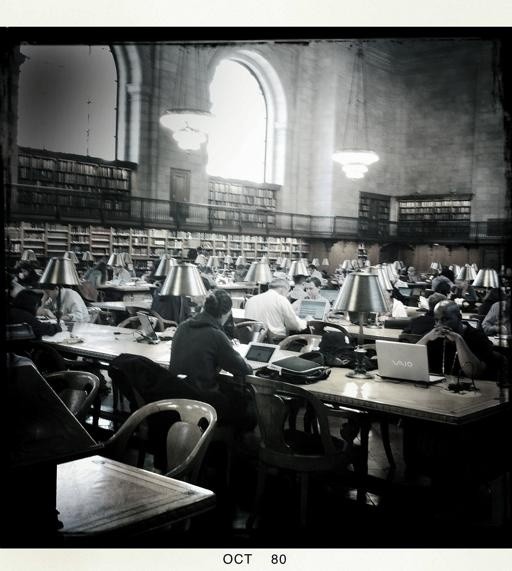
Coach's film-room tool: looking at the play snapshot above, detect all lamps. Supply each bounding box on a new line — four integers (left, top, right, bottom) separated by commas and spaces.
331, 40, 381, 181
159, 45, 217, 149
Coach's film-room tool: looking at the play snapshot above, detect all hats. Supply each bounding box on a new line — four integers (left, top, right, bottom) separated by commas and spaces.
318, 332, 355, 349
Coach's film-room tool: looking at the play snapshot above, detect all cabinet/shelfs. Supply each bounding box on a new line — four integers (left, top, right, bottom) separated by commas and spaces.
0, 146, 309, 260
358, 191, 475, 239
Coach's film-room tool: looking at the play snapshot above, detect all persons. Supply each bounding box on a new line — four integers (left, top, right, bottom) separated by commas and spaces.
113, 264, 131, 282
75, 260, 110, 308
168, 289, 267, 450
39, 282, 91, 323
7, 289, 99, 376
480, 299, 511, 336
197, 246, 210, 257
290, 276, 332, 314
478, 288, 505, 316
14, 263, 38, 287
454, 279, 476, 303
185, 248, 198, 264
413, 299, 511, 383
428, 283, 452, 311
403, 292, 467, 339
244, 277, 308, 337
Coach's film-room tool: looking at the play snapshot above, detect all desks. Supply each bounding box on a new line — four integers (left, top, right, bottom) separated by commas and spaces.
11, 242, 512, 549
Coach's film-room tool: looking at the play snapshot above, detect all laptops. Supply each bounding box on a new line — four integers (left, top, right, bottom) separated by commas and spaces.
374, 340, 446, 384
242, 342, 281, 369
137, 312, 158, 343
297, 300, 328, 321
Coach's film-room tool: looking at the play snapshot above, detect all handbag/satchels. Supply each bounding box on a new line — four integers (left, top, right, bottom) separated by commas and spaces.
255, 354, 332, 384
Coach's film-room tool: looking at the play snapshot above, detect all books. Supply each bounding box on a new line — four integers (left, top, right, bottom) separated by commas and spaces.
209, 183, 277, 222
12, 155, 131, 220
4, 223, 309, 265
356, 193, 469, 238
